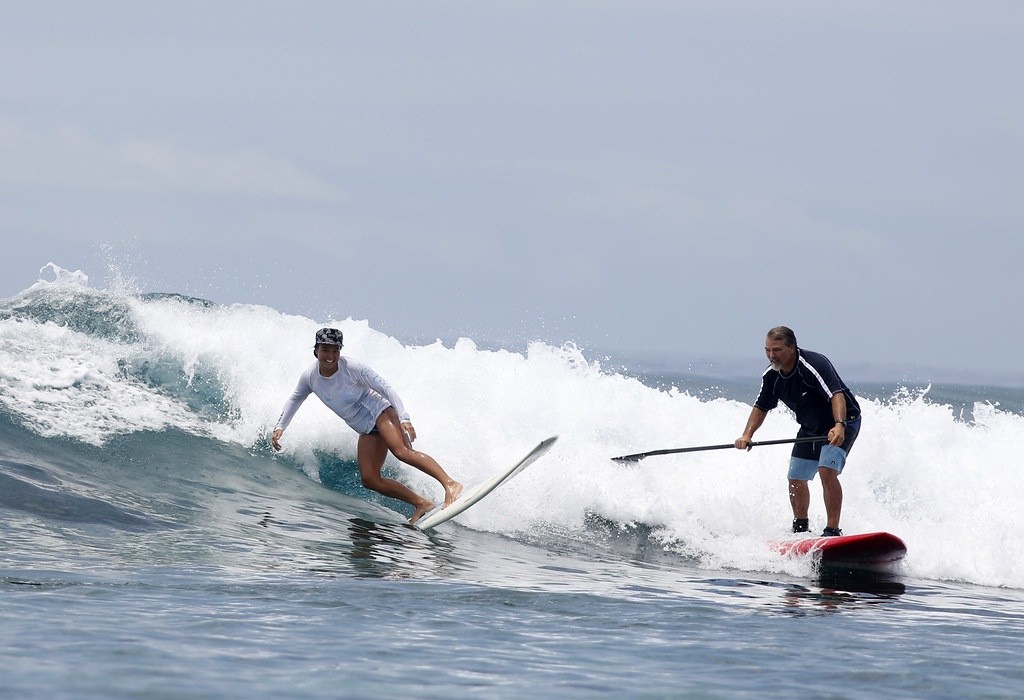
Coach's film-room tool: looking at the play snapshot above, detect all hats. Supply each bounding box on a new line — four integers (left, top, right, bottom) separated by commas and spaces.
315, 328, 343, 346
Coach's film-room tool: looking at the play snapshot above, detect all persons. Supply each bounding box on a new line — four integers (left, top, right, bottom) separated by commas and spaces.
271, 328, 462, 524
734, 326, 862, 536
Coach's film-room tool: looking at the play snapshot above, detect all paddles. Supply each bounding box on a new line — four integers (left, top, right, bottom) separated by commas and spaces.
610, 435, 831, 470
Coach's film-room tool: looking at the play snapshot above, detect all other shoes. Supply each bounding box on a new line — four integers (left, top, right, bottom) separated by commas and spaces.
820, 527, 842, 536
792, 518, 809, 533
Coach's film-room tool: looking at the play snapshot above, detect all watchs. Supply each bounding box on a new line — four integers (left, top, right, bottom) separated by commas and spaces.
835, 419, 847, 427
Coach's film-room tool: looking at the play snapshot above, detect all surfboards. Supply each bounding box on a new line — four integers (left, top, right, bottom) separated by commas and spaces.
409, 432, 560, 534
765, 530, 909, 570
